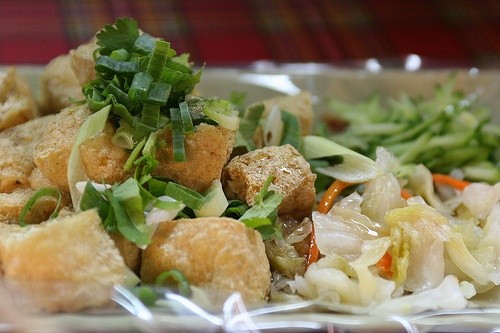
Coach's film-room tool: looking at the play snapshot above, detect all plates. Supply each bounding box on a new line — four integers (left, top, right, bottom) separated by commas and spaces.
0, 303, 500, 332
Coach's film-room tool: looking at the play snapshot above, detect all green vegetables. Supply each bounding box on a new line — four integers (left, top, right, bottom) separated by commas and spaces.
313, 75, 500, 185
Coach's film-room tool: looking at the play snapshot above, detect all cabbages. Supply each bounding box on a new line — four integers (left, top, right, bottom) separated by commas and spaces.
294, 149, 499, 319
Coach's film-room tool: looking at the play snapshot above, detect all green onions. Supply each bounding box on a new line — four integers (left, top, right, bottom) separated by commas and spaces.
17, 14, 380, 306
